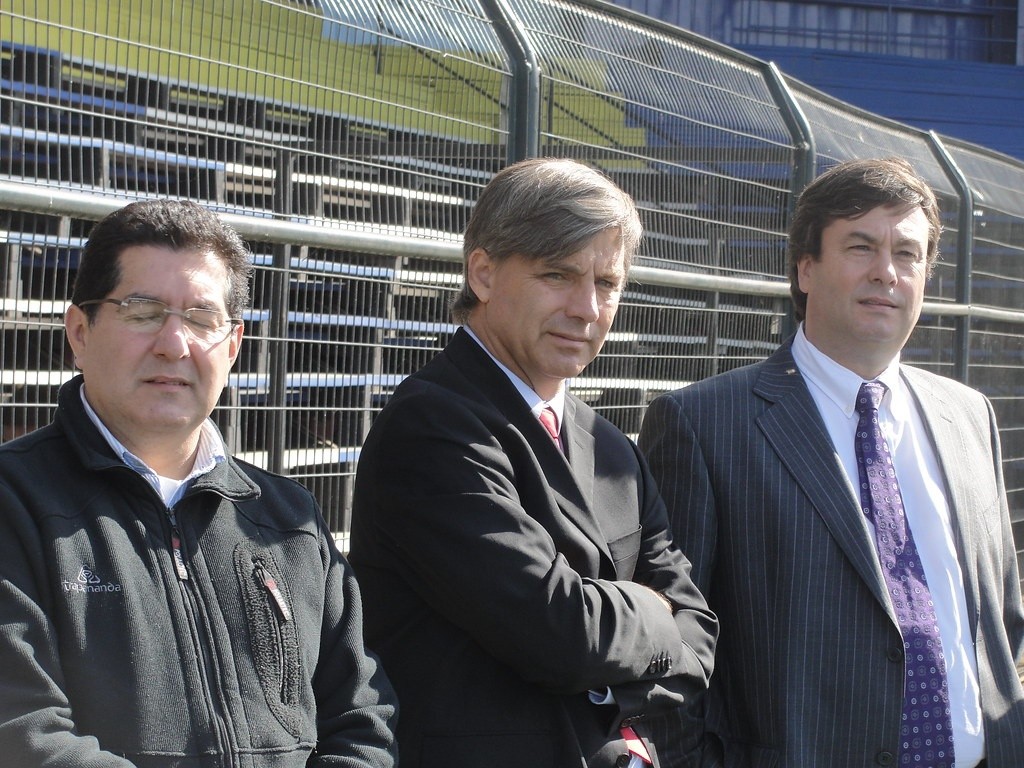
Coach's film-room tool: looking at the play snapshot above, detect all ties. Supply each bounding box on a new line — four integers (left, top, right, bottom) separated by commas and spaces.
854, 381, 956, 768
538, 407, 650, 765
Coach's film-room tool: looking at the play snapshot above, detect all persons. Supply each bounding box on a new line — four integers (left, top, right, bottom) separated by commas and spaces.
350, 156, 717, 768
0, 202, 410, 768
641, 160, 1022, 768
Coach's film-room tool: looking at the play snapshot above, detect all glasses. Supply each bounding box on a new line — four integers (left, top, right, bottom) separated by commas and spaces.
78, 297, 243, 341
